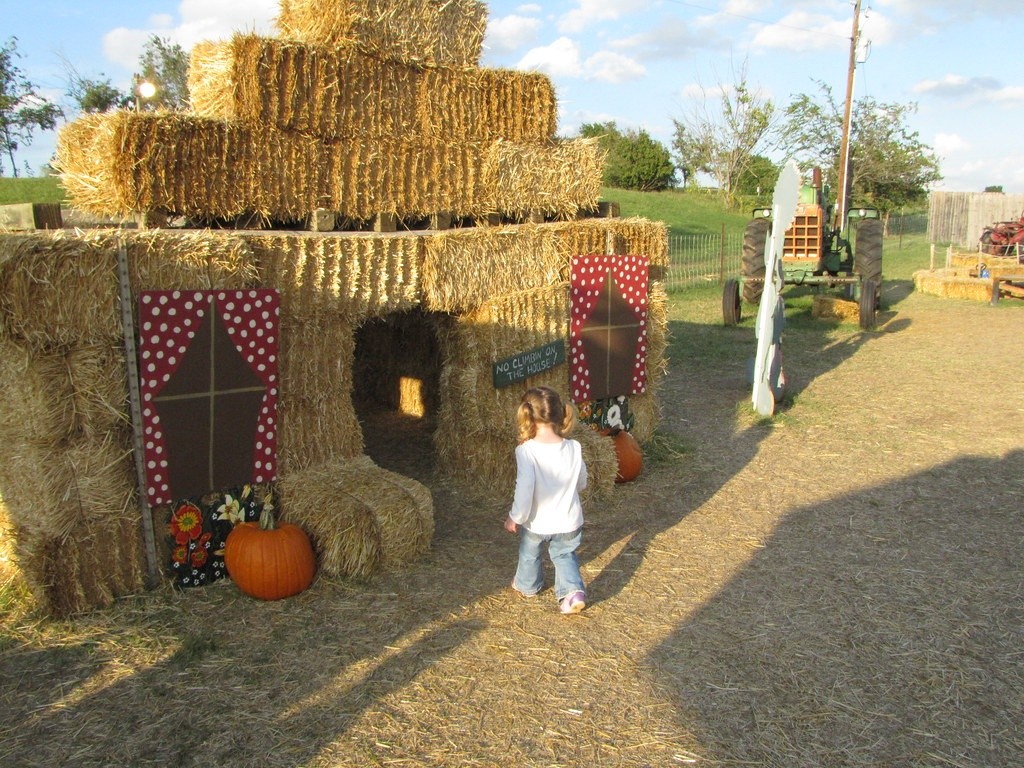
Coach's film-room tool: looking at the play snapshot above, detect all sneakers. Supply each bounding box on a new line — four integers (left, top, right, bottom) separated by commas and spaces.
560, 590, 586, 613
511, 575, 537, 597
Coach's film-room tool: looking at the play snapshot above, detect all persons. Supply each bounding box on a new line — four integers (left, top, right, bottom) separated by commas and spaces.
503, 384, 589, 615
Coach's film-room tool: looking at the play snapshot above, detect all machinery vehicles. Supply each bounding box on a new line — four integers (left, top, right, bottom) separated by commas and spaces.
723, 167, 885, 330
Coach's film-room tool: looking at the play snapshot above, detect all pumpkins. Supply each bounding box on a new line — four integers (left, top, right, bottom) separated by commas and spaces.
599, 427, 643, 483
223, 494, 316, 601
